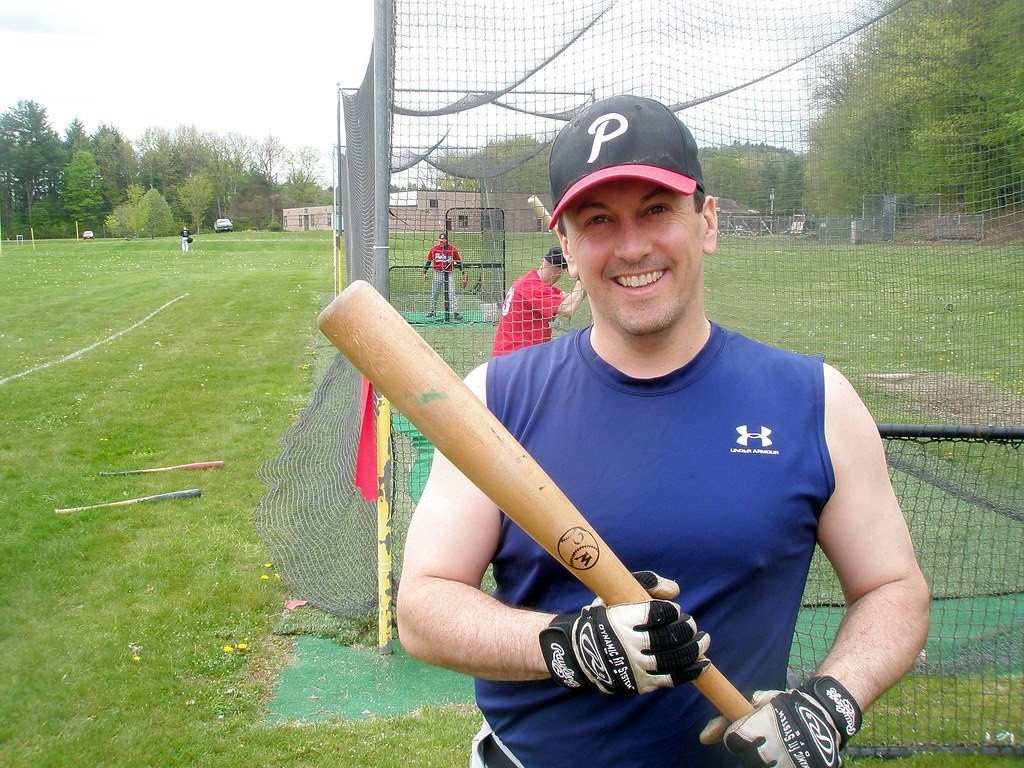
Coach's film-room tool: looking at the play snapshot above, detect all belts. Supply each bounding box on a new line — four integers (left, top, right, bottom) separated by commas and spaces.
477, 734, 520, 768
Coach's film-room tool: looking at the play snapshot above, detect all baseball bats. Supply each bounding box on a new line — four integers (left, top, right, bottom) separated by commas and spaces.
54, 488, 201, 514
98, 460, 225, 477
528, 194, 558, 235
317, 278, 757, 722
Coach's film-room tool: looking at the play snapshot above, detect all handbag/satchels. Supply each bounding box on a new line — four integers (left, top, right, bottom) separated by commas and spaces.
187, 236, 193, 243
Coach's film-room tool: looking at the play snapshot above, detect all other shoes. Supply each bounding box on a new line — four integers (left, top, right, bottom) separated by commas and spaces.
454, 313, 463, 320
426, 311, 434, 317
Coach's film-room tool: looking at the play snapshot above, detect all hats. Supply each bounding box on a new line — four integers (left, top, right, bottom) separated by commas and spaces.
545, 246, 568, 268
438, 233, 447, 242
549, 94, 706, 232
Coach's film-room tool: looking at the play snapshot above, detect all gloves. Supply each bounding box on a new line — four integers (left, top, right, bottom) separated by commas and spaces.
698, 675, 862, 768
539, 571, 710, 697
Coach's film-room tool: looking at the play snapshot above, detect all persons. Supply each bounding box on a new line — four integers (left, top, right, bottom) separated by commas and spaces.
396, 97, 931, 768
493, 245, 586, 357
422, 233, 468, 319
180, 225, 191, 251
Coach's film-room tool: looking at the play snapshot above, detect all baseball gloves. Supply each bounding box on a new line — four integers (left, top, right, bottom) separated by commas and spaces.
461, 275, 470, 290
